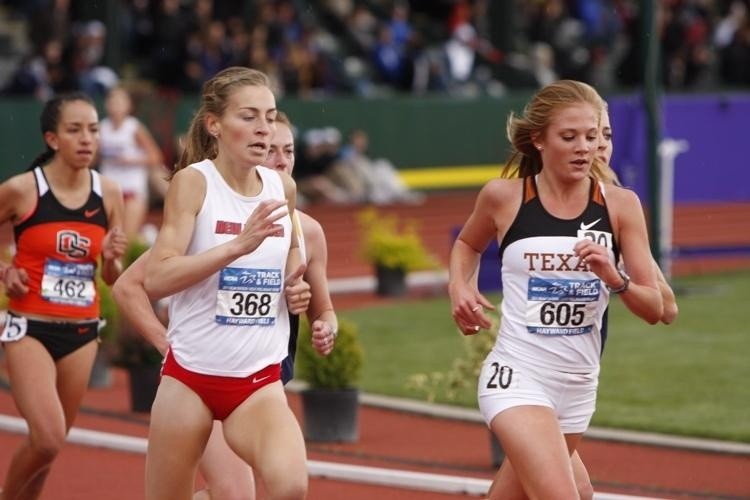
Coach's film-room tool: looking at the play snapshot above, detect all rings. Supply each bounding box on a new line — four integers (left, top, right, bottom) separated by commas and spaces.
472, 306, 479, 312
298, 293, 302, 301
322, 337, 329, 346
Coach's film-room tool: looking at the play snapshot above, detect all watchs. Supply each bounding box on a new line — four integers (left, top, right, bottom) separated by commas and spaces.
604, 268, 632, 294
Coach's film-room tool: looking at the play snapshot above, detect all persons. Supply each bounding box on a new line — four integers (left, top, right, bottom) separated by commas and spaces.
98, 84, 166, 261
108, 108, 342, 500
0, 91, 129, 498
339, 126, 431, 212
140, 61, 313, 500
461, 95, 680, 500
291, 127, 365, 203
445, 76, 666, 500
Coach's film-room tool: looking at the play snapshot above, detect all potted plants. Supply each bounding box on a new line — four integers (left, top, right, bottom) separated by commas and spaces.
295, 318, 365, 442
121, 239, 167, 412
357, 208, 439, 296
91, 258, 117, 388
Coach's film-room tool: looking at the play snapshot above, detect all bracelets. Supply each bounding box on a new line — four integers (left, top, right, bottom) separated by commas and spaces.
0, 265, 15, 282
